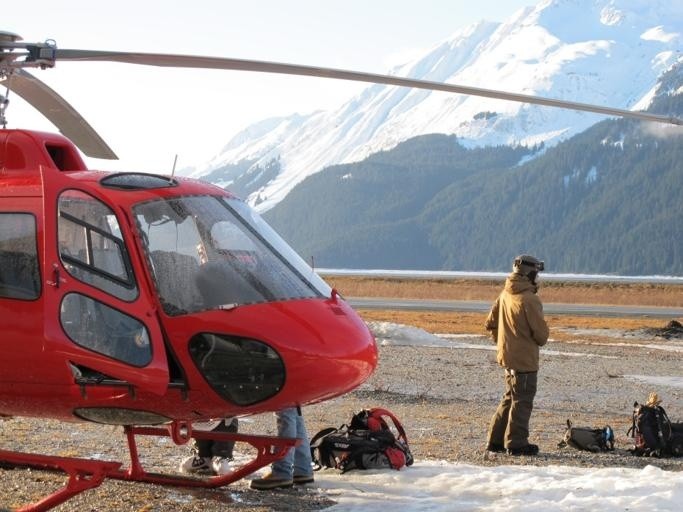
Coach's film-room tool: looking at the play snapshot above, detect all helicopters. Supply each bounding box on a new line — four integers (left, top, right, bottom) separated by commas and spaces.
0, 29, 682, 512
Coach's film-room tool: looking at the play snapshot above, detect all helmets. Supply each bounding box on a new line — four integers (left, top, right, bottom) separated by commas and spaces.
513, 256, 538, 275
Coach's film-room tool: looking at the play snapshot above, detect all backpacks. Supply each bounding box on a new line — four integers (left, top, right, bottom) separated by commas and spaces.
351, 408, 413, 468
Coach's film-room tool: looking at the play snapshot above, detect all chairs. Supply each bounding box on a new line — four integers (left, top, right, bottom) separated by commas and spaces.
148, 250, 204, 314
78, 247, 158, 366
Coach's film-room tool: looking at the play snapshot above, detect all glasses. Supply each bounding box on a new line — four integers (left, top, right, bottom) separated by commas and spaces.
514, 259, 545, 271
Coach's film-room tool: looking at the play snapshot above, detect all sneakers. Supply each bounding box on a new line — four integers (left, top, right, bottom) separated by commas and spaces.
292, 473, 315, 484
183, 455, 212, 469
252, 475, 293, 490
212, 457, 229, 473
486, 443, 505, 452
508, 444, 538, 455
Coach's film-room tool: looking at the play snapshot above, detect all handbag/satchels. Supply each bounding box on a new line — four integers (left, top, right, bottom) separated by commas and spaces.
563, 428, 607, 452
633, 402, 672, 453
319, 429, 404, 468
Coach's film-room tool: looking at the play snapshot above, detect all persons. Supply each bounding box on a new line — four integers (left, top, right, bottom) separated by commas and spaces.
484, 254, 550, 453
181, 237, 240, 475
252, 407, 314, 489
